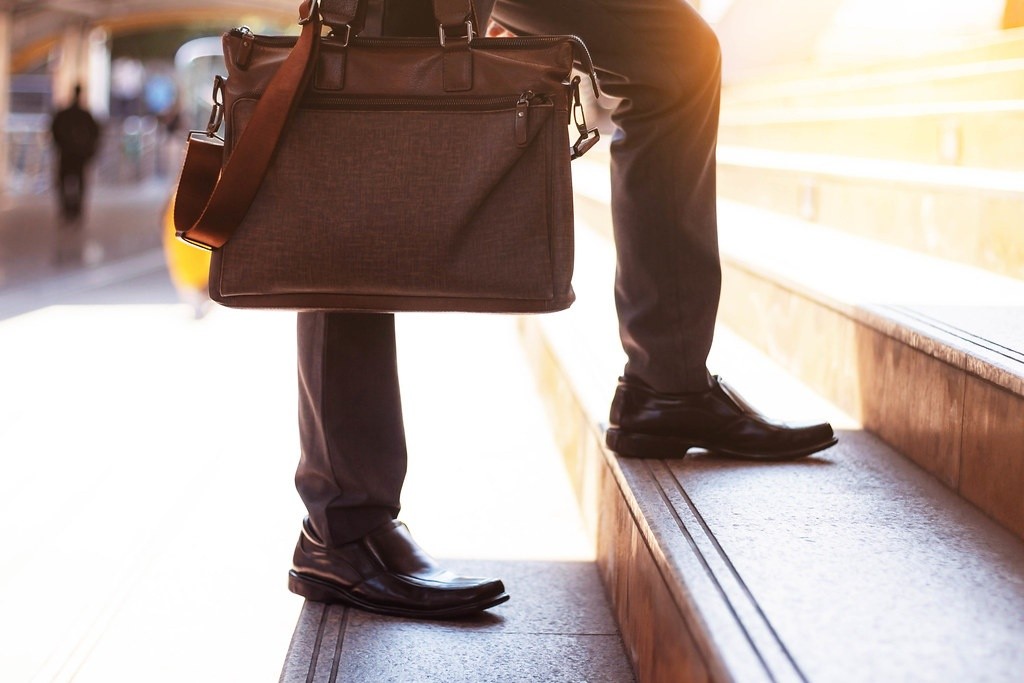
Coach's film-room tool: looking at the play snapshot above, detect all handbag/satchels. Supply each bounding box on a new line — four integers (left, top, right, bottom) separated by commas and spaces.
174, 0, 598, 313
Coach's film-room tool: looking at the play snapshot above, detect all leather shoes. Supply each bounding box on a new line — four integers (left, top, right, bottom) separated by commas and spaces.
605, 371, 839, 460
289, 515, 510, 617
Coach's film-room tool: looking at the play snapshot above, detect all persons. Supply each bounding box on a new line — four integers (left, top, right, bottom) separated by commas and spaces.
287, 0, 841, 619
50, 84, 99, 219
